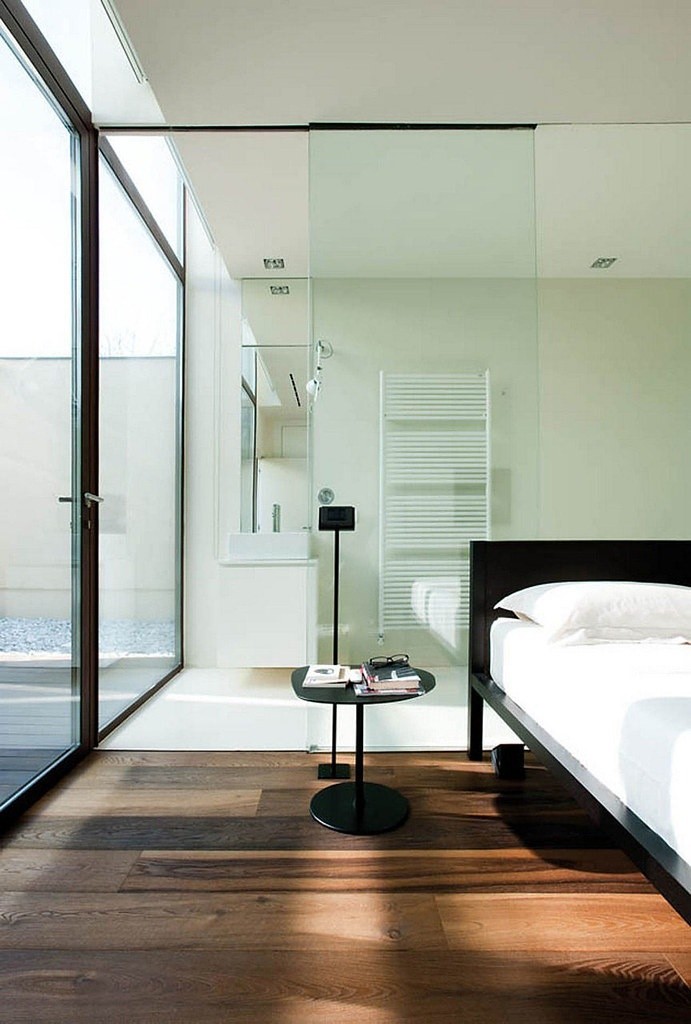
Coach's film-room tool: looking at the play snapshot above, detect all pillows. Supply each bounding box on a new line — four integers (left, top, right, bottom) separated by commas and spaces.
492, 581, 691, 646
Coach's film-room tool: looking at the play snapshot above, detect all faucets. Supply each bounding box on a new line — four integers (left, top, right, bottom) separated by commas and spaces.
272, 503, 280, 532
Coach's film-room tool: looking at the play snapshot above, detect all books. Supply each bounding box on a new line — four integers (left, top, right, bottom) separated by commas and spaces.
353, 661, 421, 696
302, 665, 351, 688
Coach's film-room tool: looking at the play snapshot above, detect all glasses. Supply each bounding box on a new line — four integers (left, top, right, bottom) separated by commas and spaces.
369, 654, 409, 670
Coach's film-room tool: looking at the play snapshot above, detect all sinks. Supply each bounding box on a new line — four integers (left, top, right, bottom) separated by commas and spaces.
229, 532, 311, 559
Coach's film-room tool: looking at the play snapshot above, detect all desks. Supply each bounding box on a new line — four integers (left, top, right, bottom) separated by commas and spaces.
291, 664, 435, 835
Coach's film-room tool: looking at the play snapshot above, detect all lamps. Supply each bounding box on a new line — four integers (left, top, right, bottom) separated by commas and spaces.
306, 339, 334, 402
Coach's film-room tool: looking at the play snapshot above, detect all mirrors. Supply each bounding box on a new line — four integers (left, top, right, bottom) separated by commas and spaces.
240, 277, 312, 533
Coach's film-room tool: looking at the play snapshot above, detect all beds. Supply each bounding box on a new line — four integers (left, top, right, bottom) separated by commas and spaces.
468, 540, 691, 927
